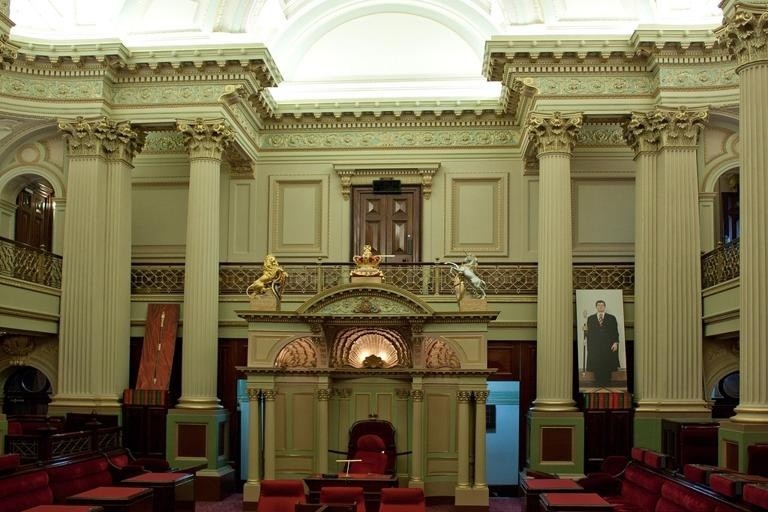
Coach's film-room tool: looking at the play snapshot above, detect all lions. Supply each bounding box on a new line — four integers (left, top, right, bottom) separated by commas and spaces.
244, 254, 290, 298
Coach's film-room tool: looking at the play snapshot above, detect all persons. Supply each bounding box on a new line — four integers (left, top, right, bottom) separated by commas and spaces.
582, 299, 620, 388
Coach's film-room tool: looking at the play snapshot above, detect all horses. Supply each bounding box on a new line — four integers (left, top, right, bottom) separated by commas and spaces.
444, 250, 486, 299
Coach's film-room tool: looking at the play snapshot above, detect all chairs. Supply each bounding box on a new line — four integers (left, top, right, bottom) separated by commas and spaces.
319, 486, 366, 512
1, 413, 196, 511
256, 479, 308, 512
344, 420, 398, 475
519, 448, 767, 511
379, 488, 427, 512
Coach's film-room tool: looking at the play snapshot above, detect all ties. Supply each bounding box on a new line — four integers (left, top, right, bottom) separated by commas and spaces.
599, 314, 603, 326
